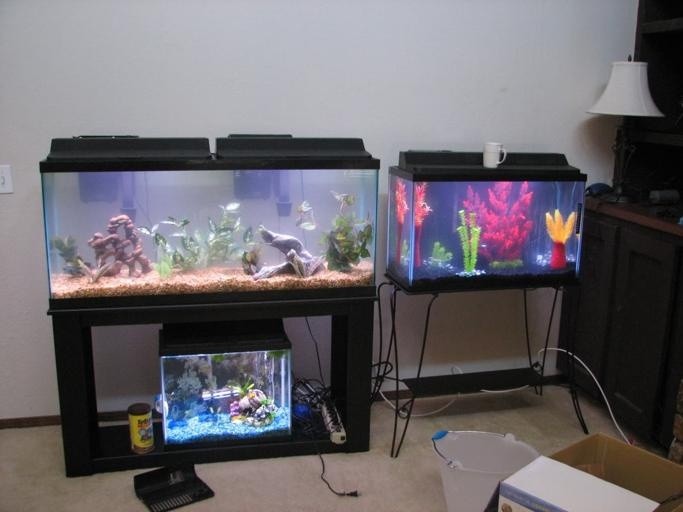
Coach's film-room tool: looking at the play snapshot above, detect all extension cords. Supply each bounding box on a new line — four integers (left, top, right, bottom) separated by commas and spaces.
321, 402, 347, 445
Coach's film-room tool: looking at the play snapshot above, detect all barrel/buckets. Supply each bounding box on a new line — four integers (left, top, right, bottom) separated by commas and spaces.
431, 427, 538, 511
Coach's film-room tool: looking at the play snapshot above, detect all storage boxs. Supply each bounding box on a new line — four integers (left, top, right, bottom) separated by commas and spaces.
498, 432, 683, 512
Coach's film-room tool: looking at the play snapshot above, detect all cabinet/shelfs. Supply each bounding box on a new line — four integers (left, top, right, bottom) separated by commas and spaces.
619, 0, 683, 225
46, 293, 381, 477
555, 207, 683, 457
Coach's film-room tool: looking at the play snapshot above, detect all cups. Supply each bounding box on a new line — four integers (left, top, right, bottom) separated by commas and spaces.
482, 142, 507, 169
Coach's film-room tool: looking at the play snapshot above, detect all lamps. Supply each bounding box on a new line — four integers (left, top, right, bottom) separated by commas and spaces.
584, 53, 665, 203
